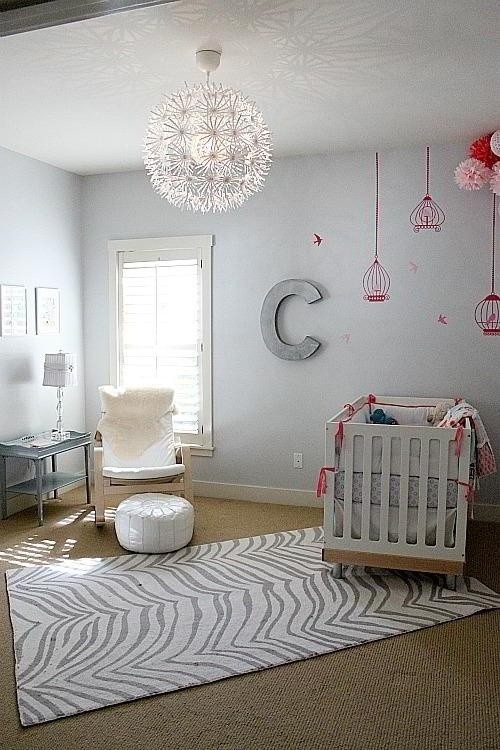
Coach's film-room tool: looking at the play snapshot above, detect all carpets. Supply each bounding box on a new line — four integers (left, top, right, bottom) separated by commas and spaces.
5, 527, 500, 729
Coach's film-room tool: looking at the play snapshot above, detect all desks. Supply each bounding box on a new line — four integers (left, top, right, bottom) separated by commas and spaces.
1, 428, 92, 527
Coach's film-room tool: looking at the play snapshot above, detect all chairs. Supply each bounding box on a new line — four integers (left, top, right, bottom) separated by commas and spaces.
94, 384, 198, 528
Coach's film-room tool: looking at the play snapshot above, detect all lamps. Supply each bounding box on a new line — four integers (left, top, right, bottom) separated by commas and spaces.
140, 44, 276, 219
41, 348, 79, 442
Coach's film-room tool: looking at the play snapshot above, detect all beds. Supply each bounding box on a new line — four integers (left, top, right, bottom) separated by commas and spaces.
321, 393, 476, 591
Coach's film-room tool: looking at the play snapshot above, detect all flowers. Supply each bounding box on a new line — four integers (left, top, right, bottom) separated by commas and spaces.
453, 128, 500, 197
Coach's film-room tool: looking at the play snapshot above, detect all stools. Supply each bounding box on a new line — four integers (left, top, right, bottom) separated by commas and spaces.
114, 492, 196, 554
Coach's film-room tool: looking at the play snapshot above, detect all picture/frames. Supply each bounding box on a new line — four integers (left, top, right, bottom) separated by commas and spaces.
0, 283, 28, 339
34, 286, 61, 337
